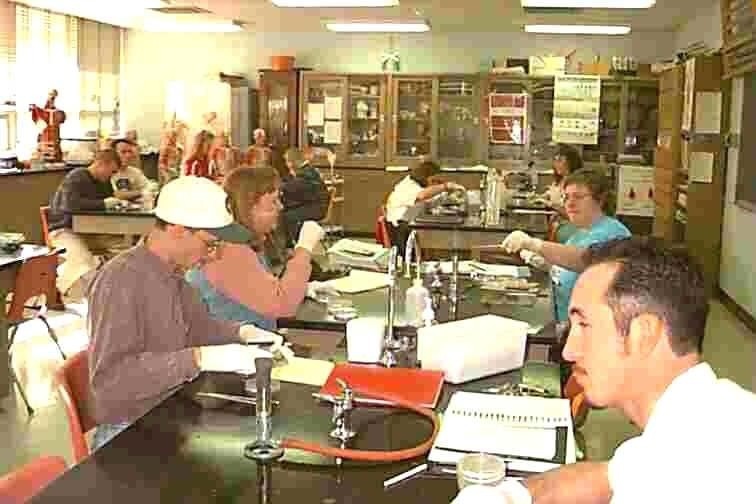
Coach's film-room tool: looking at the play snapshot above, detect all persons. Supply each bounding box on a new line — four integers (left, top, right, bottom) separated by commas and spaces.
528, 142, 587, 240
499, 166, 631, 436
46, 149, 126, 299
383, 157, 467, 263
183, 164, 326, 331
85, 174, 284, 458
448, 233, 756, 504
104, 138, 157, 259
277, 146, 331, 249
183, 128, 223, 183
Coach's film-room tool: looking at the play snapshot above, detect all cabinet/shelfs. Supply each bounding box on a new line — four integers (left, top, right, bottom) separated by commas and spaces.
658, 56, 732, 301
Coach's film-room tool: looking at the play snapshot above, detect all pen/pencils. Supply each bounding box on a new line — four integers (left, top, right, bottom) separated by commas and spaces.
469, 262, 487, 273
382, 461, 432, 488
239, 340, 293, 346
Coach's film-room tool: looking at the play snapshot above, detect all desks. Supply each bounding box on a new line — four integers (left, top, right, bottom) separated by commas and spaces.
65, 208, 156, 253
408, 200, 549, 266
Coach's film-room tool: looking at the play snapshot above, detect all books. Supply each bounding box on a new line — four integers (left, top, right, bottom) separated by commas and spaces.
428, 386, 579, 475
328, 235, 394, 296
310, 360, 449, 417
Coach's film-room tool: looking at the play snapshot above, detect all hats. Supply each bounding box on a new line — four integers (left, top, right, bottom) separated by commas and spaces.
154, 175, 258, 242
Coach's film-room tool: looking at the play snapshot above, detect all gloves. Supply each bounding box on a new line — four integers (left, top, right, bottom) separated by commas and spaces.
294, 221, 325, 254
450, 479, 533, 504
446, 183, 466, 194
500, 230, 544, 257
194, 324, 284, 374
103, 197, 121, 209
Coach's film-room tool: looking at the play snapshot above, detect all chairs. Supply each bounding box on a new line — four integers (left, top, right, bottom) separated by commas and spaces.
0, 205, 96, 504
377, 207, 392, 250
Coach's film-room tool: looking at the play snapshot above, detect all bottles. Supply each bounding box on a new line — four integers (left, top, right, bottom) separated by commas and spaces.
403, 276, 431, 323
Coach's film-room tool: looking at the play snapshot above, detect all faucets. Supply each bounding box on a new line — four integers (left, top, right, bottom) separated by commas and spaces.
383, 245, 400, 352
401, 229, 423, 283
